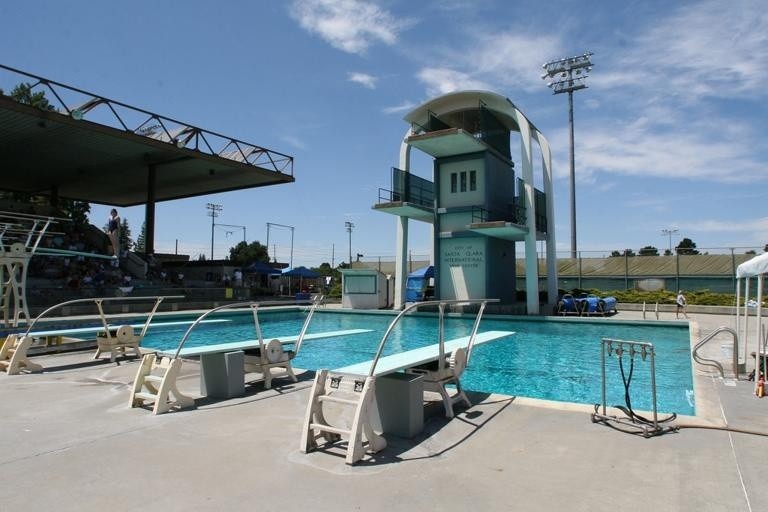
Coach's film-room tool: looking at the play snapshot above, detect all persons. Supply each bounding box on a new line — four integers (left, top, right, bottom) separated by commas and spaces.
27, 223, 309, 305
675, 290, 689, 318
106, 209, 122, 259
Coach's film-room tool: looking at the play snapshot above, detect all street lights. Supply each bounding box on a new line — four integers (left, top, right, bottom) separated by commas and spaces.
661, 226, 679, 253
207, 203, 223, 259
343, 221, 355, 262
541, 52, 595, 258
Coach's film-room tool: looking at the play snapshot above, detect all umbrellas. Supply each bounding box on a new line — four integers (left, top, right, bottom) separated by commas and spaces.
281, 265, 320, 293
